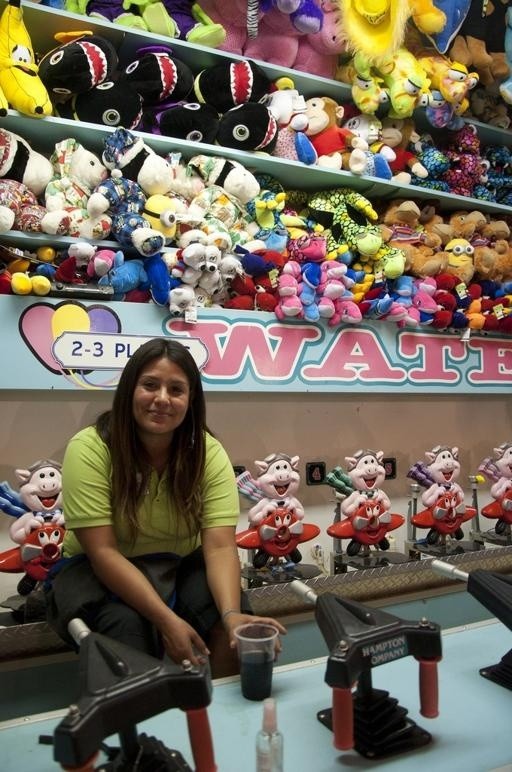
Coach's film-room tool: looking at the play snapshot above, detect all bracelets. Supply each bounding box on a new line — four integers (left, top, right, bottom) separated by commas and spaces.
221, 608, 240, 622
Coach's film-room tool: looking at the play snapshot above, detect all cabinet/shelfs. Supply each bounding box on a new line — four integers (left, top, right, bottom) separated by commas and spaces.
0, 0, 512, 338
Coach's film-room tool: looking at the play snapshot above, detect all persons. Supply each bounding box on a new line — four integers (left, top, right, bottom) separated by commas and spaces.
46, 339, 288, 685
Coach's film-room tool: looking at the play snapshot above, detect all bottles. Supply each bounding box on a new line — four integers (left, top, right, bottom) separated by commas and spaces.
254, 698, 284, 772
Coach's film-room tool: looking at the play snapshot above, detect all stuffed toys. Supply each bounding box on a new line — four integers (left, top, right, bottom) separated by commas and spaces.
0, 0, 512, 340
0, 441, 511, 594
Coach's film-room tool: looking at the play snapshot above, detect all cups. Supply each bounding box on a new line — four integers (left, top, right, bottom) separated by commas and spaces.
235, 623, 278, 700
